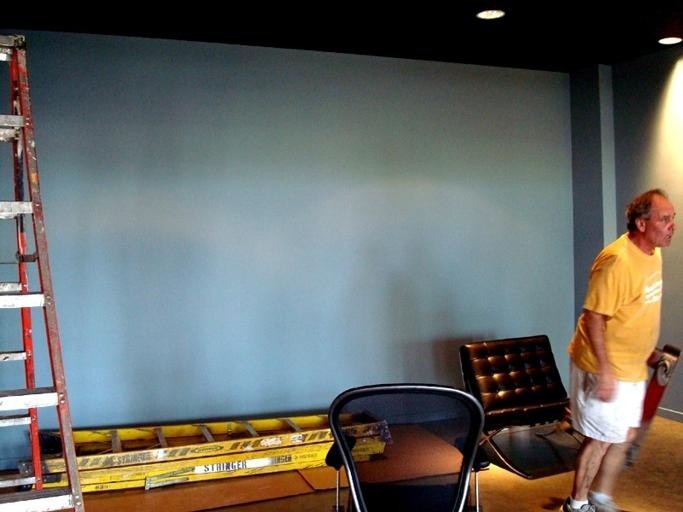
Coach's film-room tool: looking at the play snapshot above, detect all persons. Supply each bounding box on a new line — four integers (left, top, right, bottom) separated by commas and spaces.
557, 188, 677, 512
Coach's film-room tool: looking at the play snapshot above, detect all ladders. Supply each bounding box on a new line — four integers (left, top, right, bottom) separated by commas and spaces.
0, 34, 85, 512
17, 412, 392, 493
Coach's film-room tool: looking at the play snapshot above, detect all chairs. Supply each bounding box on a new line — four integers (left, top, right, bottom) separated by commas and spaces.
459, 334, 591, 512
325, 383, 485, 512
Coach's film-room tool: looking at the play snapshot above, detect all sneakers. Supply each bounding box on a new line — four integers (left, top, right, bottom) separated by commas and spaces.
560, 494, 627, 511
